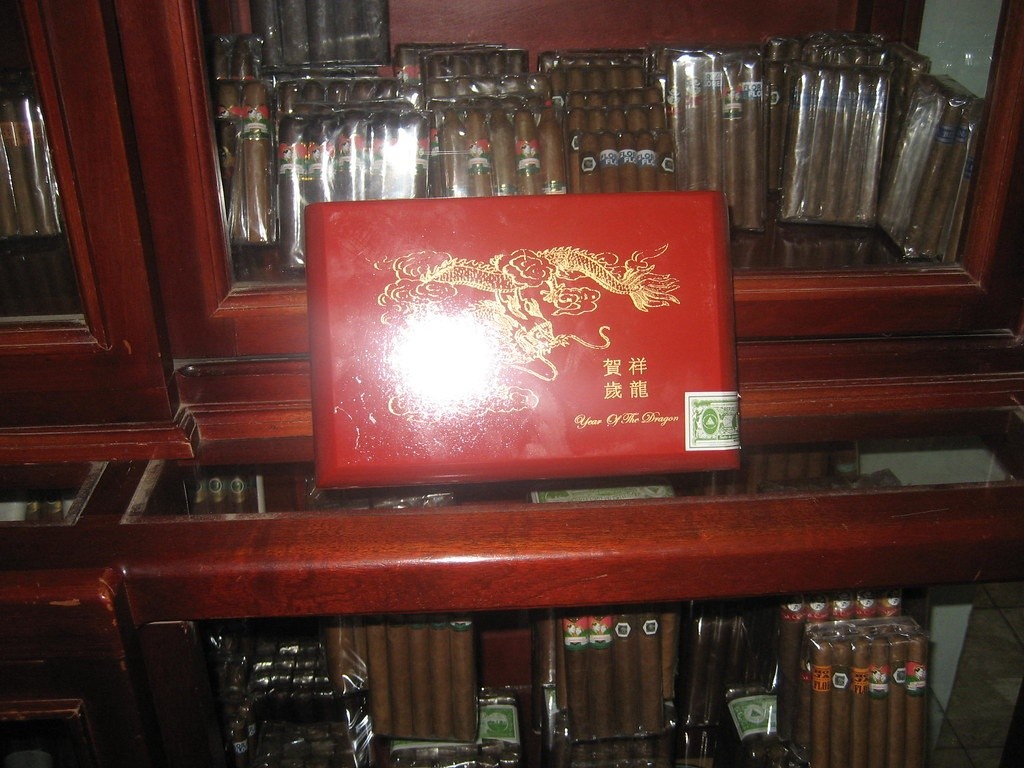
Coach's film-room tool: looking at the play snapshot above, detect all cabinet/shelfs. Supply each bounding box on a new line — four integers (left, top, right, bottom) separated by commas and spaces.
0, 0, 1024, 767
